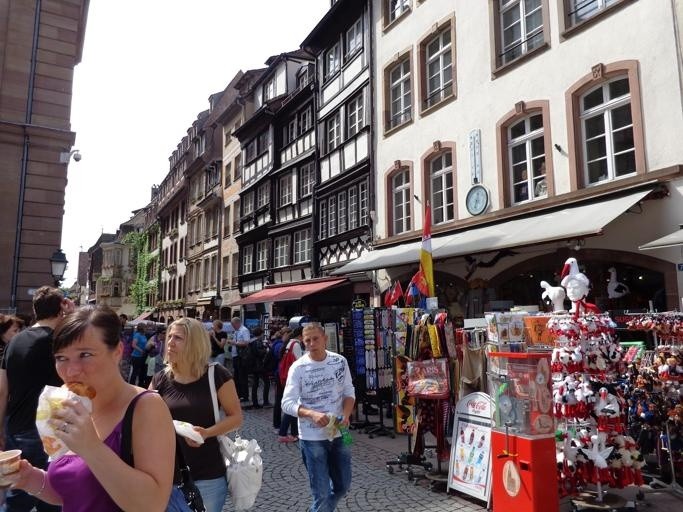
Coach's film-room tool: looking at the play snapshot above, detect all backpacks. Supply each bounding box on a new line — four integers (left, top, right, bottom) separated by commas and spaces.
279, 341, 300, 384
263, 342, 280, 375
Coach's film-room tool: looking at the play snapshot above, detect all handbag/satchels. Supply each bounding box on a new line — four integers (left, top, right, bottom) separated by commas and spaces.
406, 349, 451, 400
178, 465, 207, 512
217, 434, 263, 511
236, 345, 249, 360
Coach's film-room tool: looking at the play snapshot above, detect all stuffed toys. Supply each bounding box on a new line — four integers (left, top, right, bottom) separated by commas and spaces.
543, 305, 682, 502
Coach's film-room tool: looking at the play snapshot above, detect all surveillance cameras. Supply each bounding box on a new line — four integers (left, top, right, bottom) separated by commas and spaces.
73, 154, 81, 162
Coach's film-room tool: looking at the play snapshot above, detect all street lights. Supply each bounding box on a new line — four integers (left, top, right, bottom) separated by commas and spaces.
46, 247, 67, 288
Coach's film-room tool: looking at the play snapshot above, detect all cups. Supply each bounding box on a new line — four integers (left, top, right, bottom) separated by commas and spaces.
0, 449, 22, 477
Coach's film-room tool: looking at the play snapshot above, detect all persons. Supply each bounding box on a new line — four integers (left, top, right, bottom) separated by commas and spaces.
534, 160, 548, 197
517, 170, 528, 201
564, 278, 587, 301
0, 285, 302, 511
279, 321, 357, 511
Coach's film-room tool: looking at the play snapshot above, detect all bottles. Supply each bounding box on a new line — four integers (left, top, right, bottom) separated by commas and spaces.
336, 415, 352, 445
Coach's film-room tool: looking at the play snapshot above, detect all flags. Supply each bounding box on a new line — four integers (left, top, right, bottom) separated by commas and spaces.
411, 203, 436, 297
384, 280, 417, 307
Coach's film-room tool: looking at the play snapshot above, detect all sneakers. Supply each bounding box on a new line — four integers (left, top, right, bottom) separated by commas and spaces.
273, 427, 298, 442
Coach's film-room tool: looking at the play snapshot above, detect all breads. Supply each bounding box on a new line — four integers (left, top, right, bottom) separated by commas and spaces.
66, 383, 98, 398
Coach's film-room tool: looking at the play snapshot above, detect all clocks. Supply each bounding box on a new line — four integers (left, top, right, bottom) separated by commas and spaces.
465, 184, 490, 216
